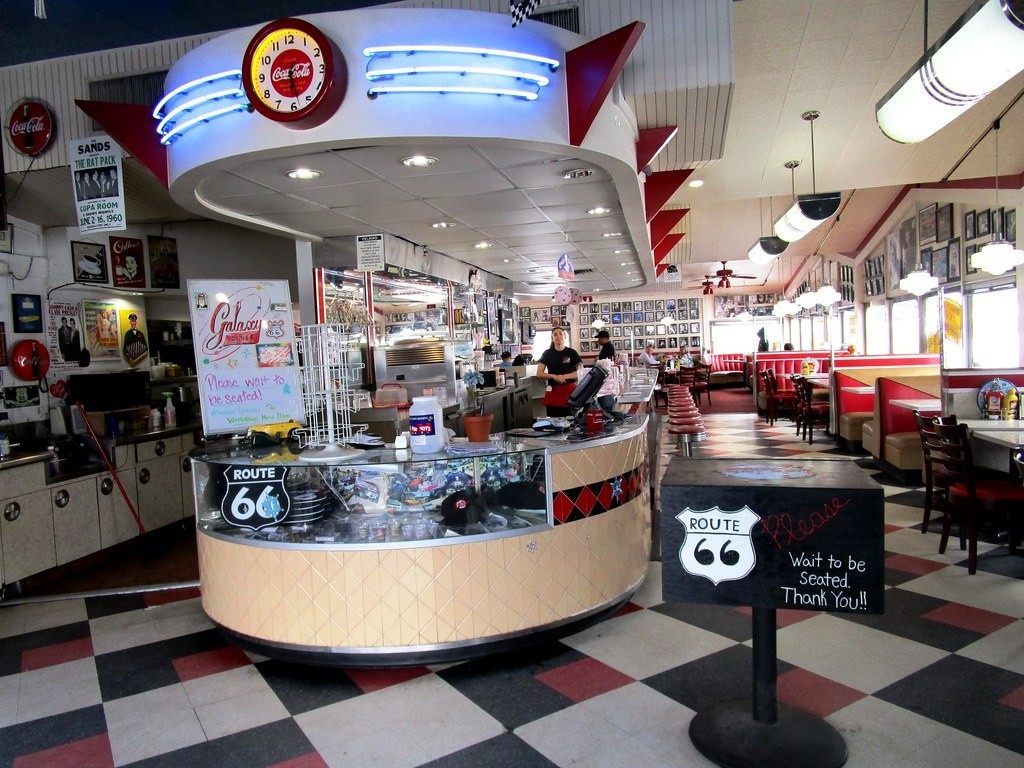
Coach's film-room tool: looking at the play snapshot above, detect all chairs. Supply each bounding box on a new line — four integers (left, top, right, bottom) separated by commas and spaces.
933, 415, 1024, 574
768, 368, 797, 422
791, 374, 830, 436
794, 378, 831, 445
913, 410, 968, 549
657, 362, 713, 407
760, 370, 801, 426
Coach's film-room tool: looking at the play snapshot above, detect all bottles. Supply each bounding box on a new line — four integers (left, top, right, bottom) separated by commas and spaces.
408, 395, 444, 454
514, 369, 519, 388
1003, 388, 1018, 419
22, 104, 34, 150
0, 434, 10, 456
803, 361, 809, 375
32, 343, 40, 377
47, 446, 59, 477
987, 391, 1002, 419
164, 396, 176, 426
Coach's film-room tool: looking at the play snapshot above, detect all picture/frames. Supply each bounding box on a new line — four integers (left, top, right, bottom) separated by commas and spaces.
0, 223, 14, 254
374, 292, 703, 359
900, 204, 1017, 290
714, 291, 774, 320
69, 240, 107, 283
0, 321, 7, 367
788, 257, 886, 319
12, 294, 42, 333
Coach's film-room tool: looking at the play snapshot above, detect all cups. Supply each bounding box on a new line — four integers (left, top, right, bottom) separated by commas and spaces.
84, 254, 101, 269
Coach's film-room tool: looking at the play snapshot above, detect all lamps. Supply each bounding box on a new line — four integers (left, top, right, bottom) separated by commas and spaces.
772, 110, 840, 245
771, 287, 844, 319
970, 121, 1024, 276
875, 0, 1024, 148
900, 200, 938, 297
747, 191, 790, 268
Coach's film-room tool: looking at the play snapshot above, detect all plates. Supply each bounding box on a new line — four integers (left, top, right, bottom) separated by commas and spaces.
78, 261, 101, 274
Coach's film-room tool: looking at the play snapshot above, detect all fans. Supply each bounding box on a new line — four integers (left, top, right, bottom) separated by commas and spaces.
684, 258, 757, 295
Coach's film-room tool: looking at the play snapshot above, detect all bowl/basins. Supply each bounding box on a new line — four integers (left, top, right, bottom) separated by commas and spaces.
152, 363, 184, 379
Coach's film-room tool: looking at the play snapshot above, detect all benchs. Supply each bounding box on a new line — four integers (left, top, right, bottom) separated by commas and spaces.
705, 348, 966, 484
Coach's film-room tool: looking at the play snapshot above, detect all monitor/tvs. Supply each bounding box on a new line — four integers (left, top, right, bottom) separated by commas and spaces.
567, 365, 608, 407
512, 354, 532, 366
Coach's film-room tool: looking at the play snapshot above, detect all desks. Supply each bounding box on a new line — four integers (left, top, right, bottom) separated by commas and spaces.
956, 410, 1024, 431
808, 379, 830, 388
976, 431, 1023, 450
841, 386, 875, 394
890, 397, 942, 409
722, 359, 746, 363
775, 372, 829, 378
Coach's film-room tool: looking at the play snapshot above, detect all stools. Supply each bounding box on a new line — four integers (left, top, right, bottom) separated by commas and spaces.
664, 385, 708, 457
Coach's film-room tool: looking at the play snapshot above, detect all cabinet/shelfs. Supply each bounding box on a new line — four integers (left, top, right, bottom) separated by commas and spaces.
150, 320, 195, 384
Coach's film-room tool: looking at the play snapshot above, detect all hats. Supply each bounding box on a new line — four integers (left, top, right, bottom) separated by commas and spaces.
500, 351, 512, 358
595, 331, 610, 338
129, 313, 137, 320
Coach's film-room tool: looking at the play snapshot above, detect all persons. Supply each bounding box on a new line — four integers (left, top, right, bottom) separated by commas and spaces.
87, 310, 117, 355
679, 346, 693, 367
638, 345, 661, 365
783, 343, 794, 351
123, 313, 148, 360
75, 169, 119, 202
594, 330, 615, 359
498, 351, 514, 366
536, 327, 583, 416
58, 317, 80, 360
